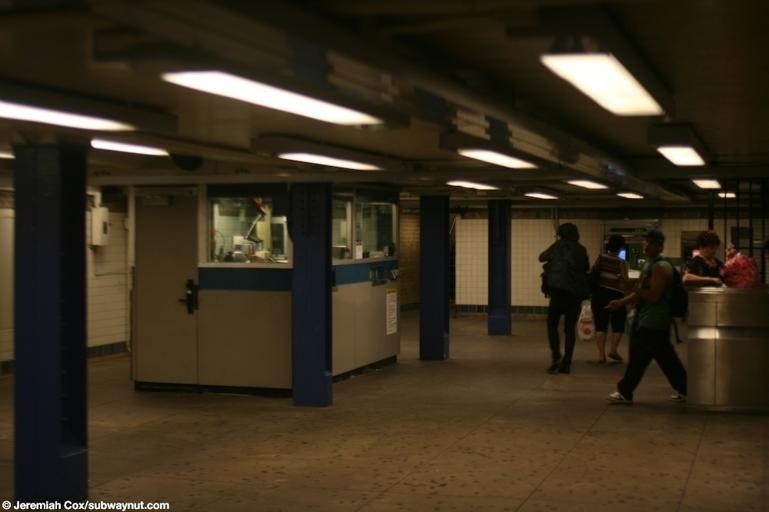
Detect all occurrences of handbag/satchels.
[577,299,596,341]
[720,248,759,289]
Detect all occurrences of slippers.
[608,352,622,361]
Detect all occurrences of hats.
[640,230,665,242]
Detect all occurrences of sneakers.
[670,394,686,402]
[605,392,634,404]
[547,361,571,373]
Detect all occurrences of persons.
[538,223,590,375]
[591,233,629,364]
[606,229,689,404]
[682,231,725,287]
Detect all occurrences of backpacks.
[647,257,688,321]
[540,257,573,299]
